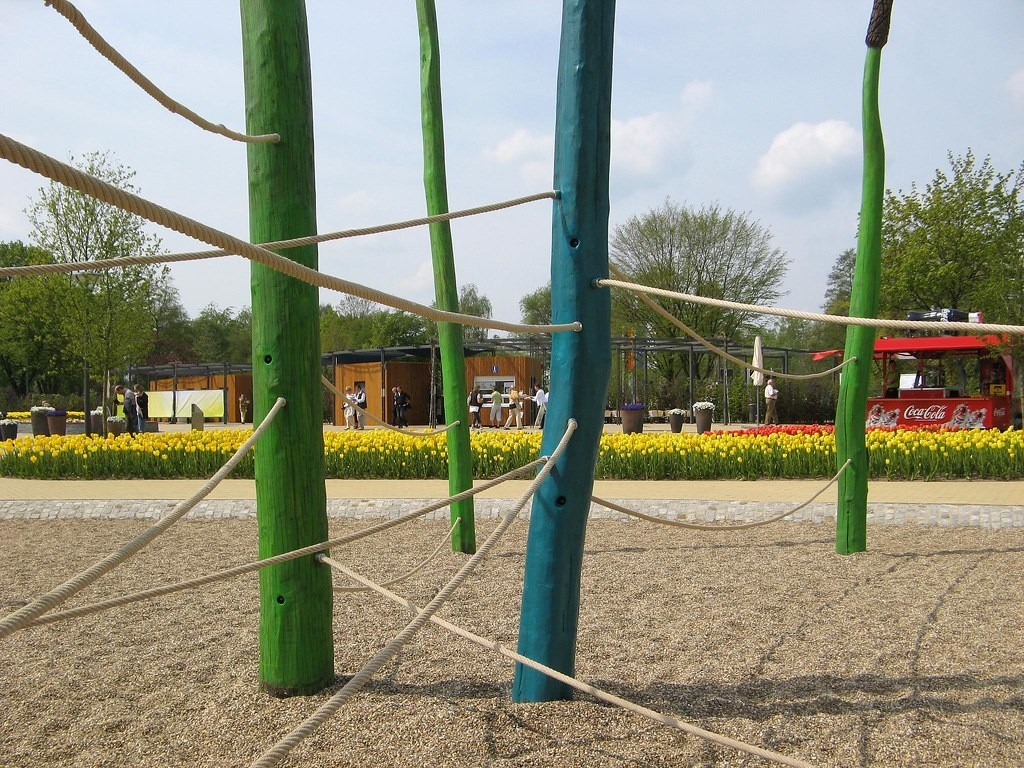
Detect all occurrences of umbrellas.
[749,336,764,425]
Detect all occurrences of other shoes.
[357,427,364,430]
[345,427,350,430]
[504,427,510,430]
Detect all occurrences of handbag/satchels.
[509,403,516,409]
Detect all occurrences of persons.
[765,379,782,426]
[391,386,411,428]
[884,360,900,398]
[134,384,148,433]
[239,394,248,424]
[343,385,367,430]
[489,387,502,428]
[504,385,550,430]
[115,385,137,433]
[467,385,483,431]
[1013,402,1022,430]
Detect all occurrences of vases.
[622,409,644,435]
[695,408,713,435]
[47,416,67,436]
[1,424,18,442]
[90,414,103,435]
[107,421,125,434]
[671,413,684,433]
[31,411,50,438]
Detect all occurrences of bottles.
[965,409,985,427]
[869,405,883,424]
[884,410,896,425]
[953,404,967,425]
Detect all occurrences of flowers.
[668,408,686,416]
[91,410,103,415]
[0,418,20,426]
[30,406,56,412]
[620,403,646,410]
[107,416,125,423]
[43,409,67,416]
[693,402,716,411]
